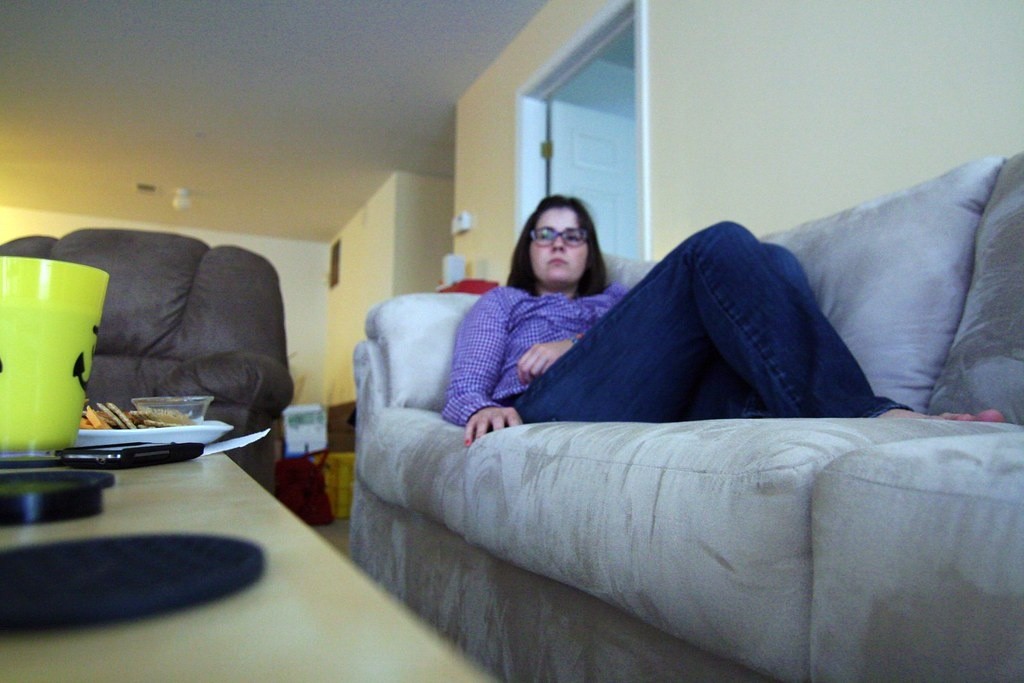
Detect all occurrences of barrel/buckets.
[0,254,111,454]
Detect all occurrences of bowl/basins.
[131,395,216,428]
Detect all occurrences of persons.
[441,194,1006,446]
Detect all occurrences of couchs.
[0,227,293,497]
[349,154,1022,682]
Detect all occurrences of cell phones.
[61,441,209,470]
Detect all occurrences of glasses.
[530,228,588,247]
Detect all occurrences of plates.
[72,418,235,447]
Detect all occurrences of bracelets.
[568,336,579,343]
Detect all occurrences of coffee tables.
[0,449,489,683]
